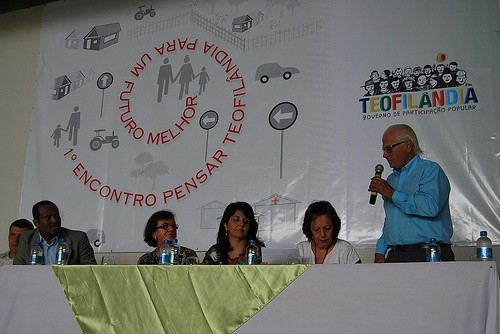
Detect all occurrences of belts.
[390,243,451,251]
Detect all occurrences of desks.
[0,260,500,334]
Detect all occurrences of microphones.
[370,164,384,204]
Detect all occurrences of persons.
[0,219,34,266]
[13,201,97,265]
[202,202,266,265]
[298,201,361,265]
[137,211,198,265]
[368,124,454,264]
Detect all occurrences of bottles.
[246,240,260,265]
[29,242,43,265]
[55,238,68,265]
[426,238,441,262]
[476,231,493,261]
[169,239,180,265]
[160,240,170,265]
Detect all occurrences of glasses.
[157,223,178,230]
[383,141,405,154]
